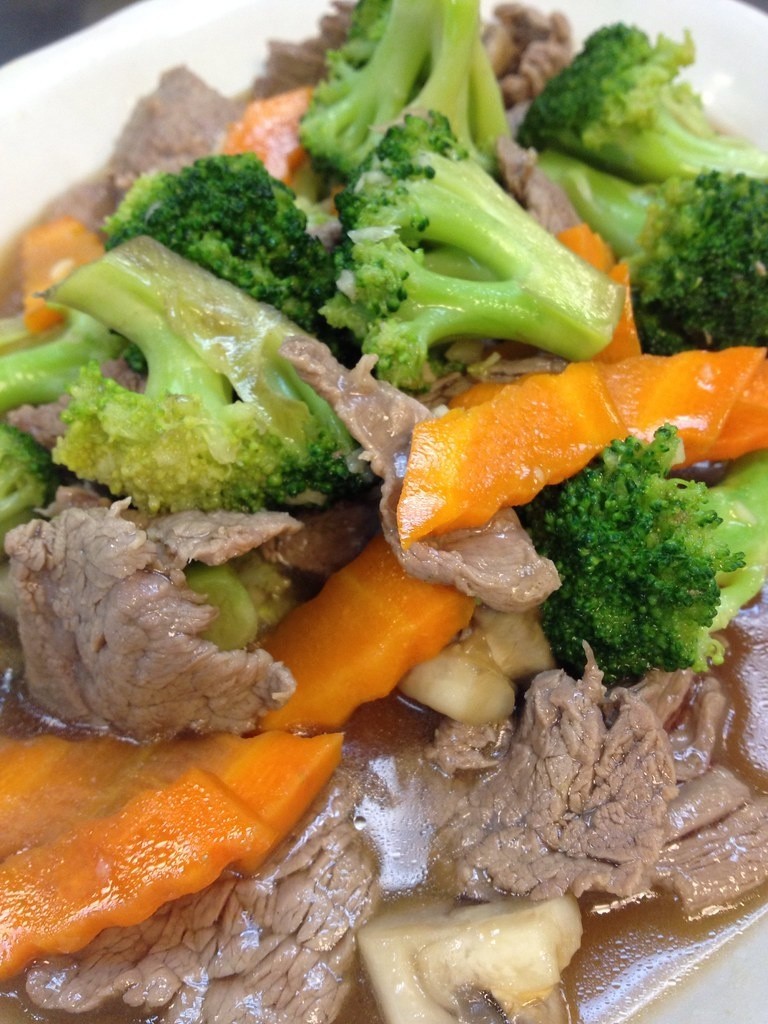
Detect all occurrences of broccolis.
[0,0,768,687]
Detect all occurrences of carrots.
[0,93,768,980]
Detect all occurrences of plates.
[0,1,768,1023]
